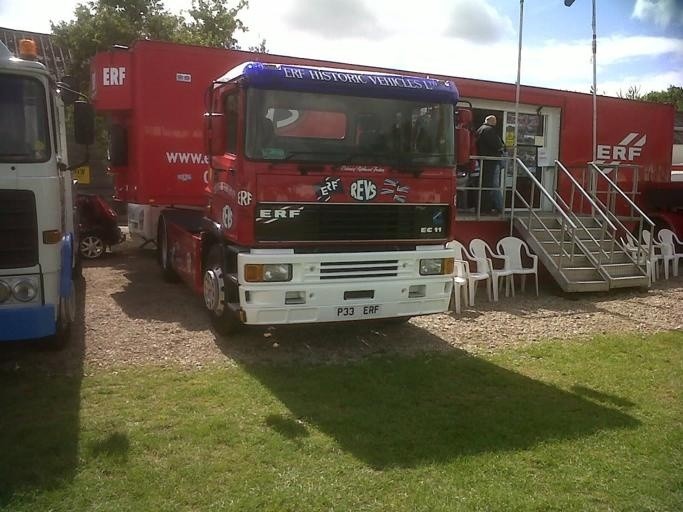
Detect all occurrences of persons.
[476,115,507,216]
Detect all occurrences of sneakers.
[463,206,500,217]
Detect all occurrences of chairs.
[619,233,656,287]
[446,239,492,307]
[416,244,468,314]
[469,238,515,301]
[658,229,683,276]
[497,237,538,296]
[642,230,677,280]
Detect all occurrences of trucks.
[0,33,85,345]
[155,60,478,335]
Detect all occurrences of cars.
[72,186,126,263]
